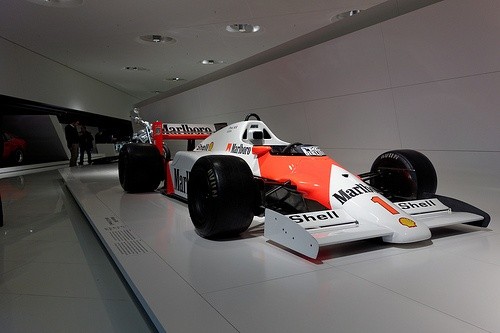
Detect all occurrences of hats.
[79,127,87,133]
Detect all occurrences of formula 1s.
[119,108,491,260]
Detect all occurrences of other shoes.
[87,161,92,165]
[78,162,83,165]
[70,164,76,167]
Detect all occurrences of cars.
[2,133,25,165]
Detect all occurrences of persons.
[95,127,105,144]
[78,124,94,166]
[65,118,80,167]
